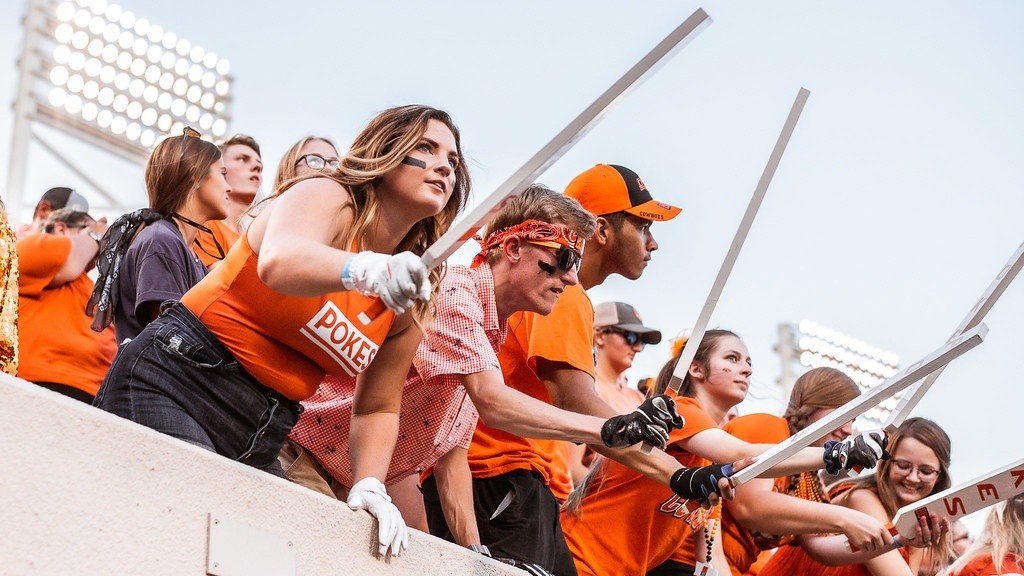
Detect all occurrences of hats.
[34,187,89,220]
[565,164,682,220]
[595,302,662,344]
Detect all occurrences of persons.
[1,104,1024,576]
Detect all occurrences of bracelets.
[893,535,902,548]
[342,255,354,290]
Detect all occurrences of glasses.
[526,239,582,274]
[180,127,201,157]
[892,458,942,482]
[296,154,339,171]
[953,531,969,543]
[604,328,647,348]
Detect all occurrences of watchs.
[88,231,101,243]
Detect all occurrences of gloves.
[341,250,431,315]
[347,476,407,557]
[601,394,684,451]
[823,429,891,475]
